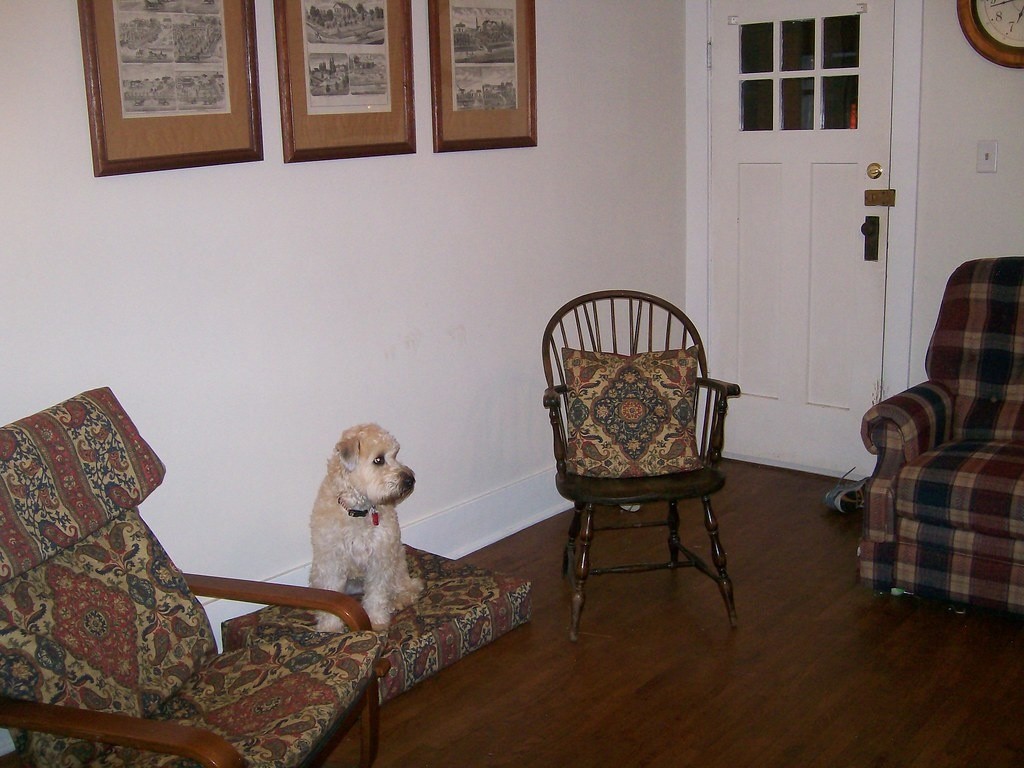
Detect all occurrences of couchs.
[857,257,1024,616]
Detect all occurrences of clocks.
[957,0,1024,68]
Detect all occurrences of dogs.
[308,423,426,632]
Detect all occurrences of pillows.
[557,345,703,477]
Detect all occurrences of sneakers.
[825,466,871,515]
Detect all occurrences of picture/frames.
[428,0,537,153]
[78,0,264,179]
[274,0,416,165]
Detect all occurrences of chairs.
[0,384,388,768]
[541,289,740,641]
